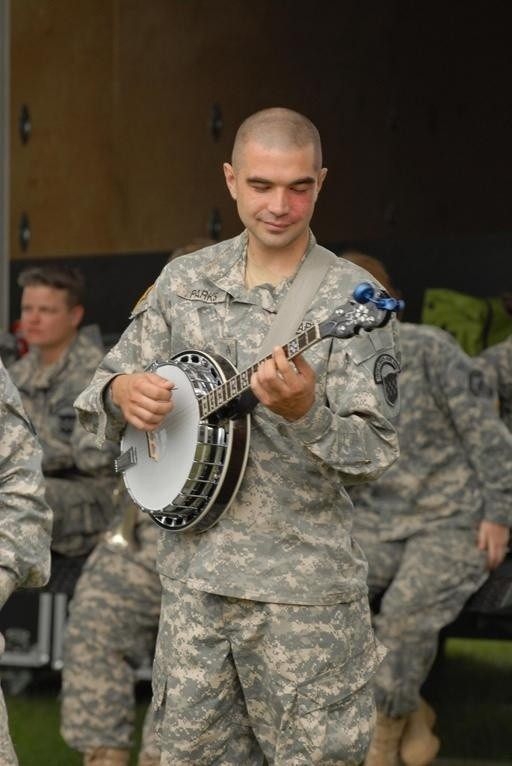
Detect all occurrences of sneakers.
[365,696,440,766]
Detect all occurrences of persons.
[338,248,512,766]
[57,497,162,766]
[468,332,512,614]
[0,357,58,766]
[9,260,117,558]
[66,104,406,766]
